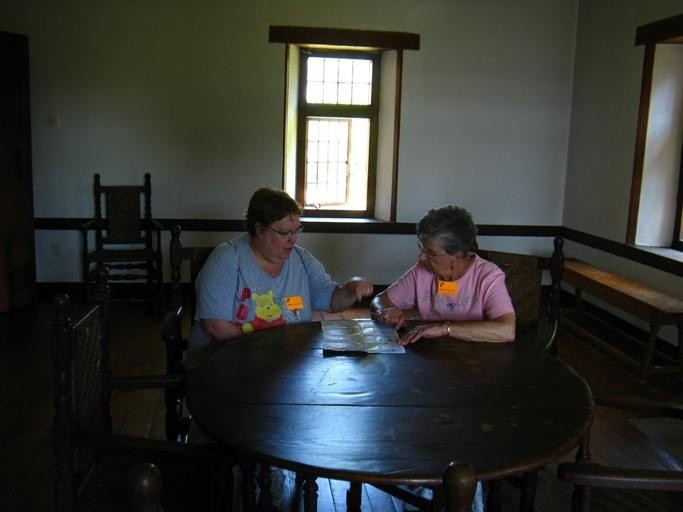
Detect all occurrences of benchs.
[556,257,681,384]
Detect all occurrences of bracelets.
[447,322,451,338]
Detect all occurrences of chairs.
[77,172,162,319]
[554,392,681,512]
[162,225,216,444]
[51,267,236,512]
[470,234,566,510]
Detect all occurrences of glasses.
[267,223,305,238]
[417,240,450,259]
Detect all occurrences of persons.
[184,189,374,372]
[370,206,516,511]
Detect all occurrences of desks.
[183,317,596,512]
